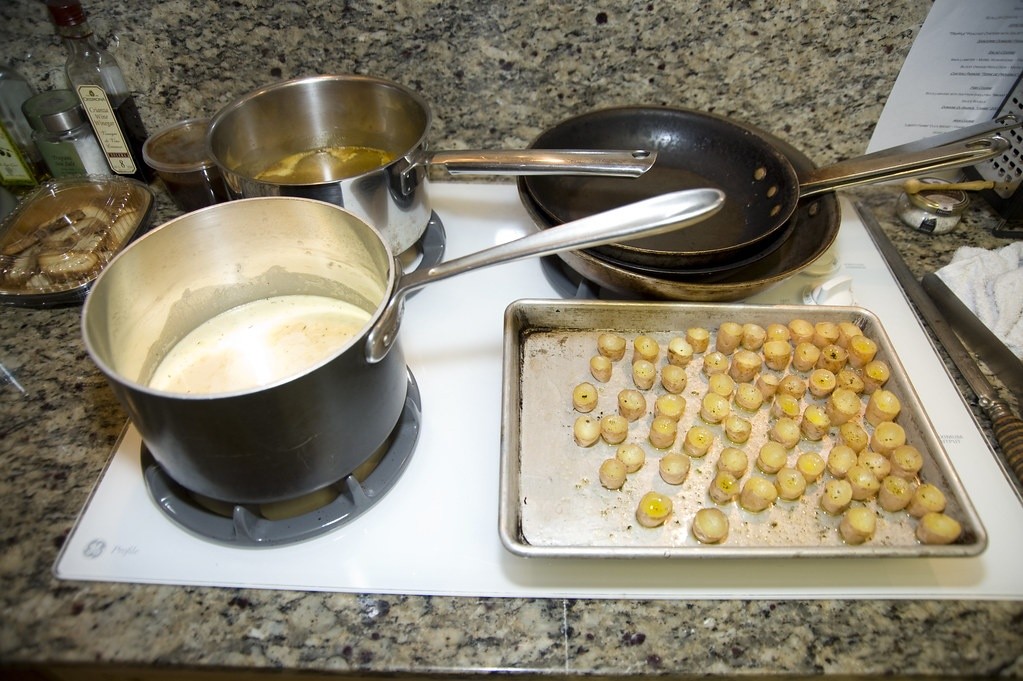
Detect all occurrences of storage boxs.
[0,169,158,309]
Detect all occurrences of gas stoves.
[49,155,1023,602]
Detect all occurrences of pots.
[205,74,658,290]
[513,105,1022,303]
[82,186,725,505]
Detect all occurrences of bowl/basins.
[142,115,227,213]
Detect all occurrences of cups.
[896,177,968,234]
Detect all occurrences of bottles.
[22,89,113,177]
[45,0,156,182]
[1,67,52,189]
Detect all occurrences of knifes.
[918,275,1023,407]
